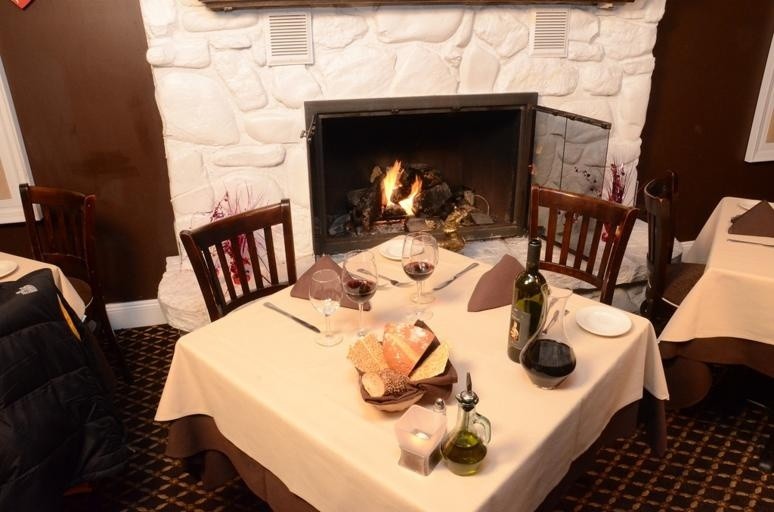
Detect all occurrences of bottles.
[507,239,551,363]
[520,282,579,390]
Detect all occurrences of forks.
[355,268,412,288]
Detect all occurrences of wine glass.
[307,267,346,348]
[343,249,379,339]
[400,231,440,322]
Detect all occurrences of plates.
[571,305,631,339]
[381,240,425,262]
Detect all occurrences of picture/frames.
[0,65,42,225]
[743,34,774,164]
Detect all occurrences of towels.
[728,201,773,248]
[467,253,539,311]
[289,255,375,313]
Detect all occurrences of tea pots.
[440,369,494,476]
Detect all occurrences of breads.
[347,320,450,397]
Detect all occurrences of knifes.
[263,300,321,333]
[430,261,481,290]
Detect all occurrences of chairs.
[638,168,705,330]
[18,181,132,384]
[179,196,299,321]
[525,182,641,304]
